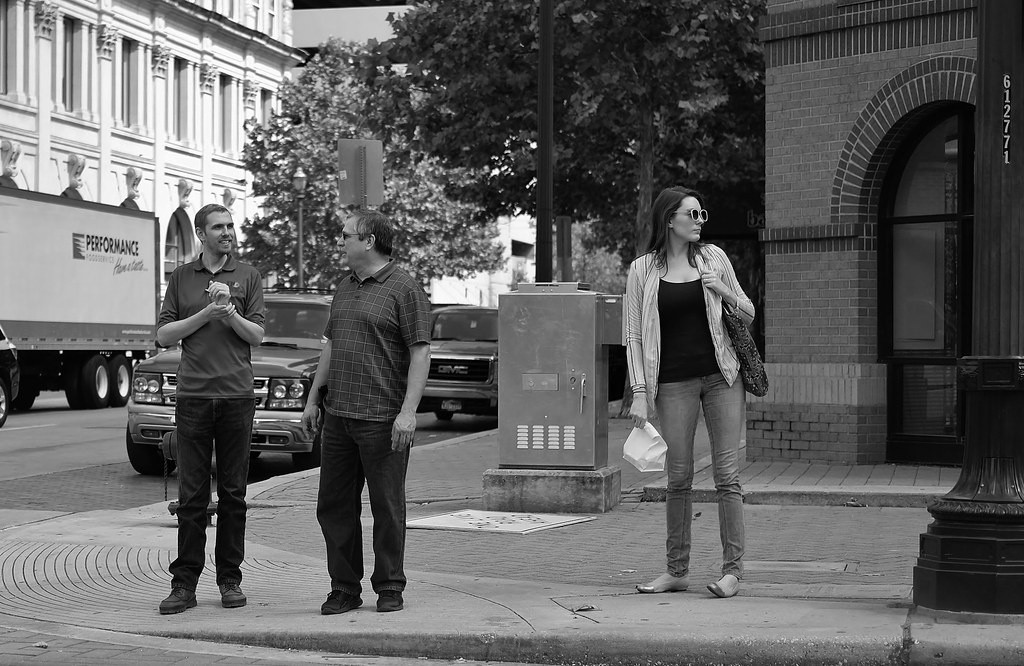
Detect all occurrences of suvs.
[125,288,335,479]
[415,304,497,420]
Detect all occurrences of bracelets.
[632,386,647,396]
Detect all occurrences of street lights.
[294,167,308,294]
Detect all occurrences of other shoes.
[635,583,688,592]
[706,574,739,598]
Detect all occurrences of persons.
[301,207,433,616]
[156,204,266,615]
[625,184,756,599]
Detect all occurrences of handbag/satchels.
[621,420,667,473]
[720,299,769,398]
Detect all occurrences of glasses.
[342,232,371,240]
[671,209,709,223]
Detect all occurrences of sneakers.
[321,590,363,614]
[219,583,247,608]
[376,590,404,612]
[159,587,197,615]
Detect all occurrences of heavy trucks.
[0,185,160,418]
